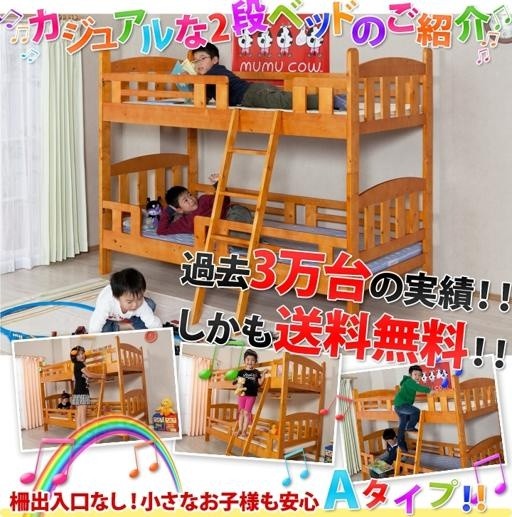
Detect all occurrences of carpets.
[1,274,323,360]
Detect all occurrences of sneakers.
[405,426,417,432]
[399,444,408,453]
[237,431,248,437]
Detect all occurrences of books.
[171,58,197,103]
[367,460,394,474]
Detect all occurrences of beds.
[92,42,435,336]
[32,337,150,443]
[203,350,328,464]
[350,371,505,479]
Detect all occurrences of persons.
[233,349,268,437]
[87,268,162,334]
[58,393,72,415]
[234,376,247,397]
[181,42,348,110]
[71,346,106,430]
[370,428,408,478]
[393,365,438,453]
[157,173,269,253]
